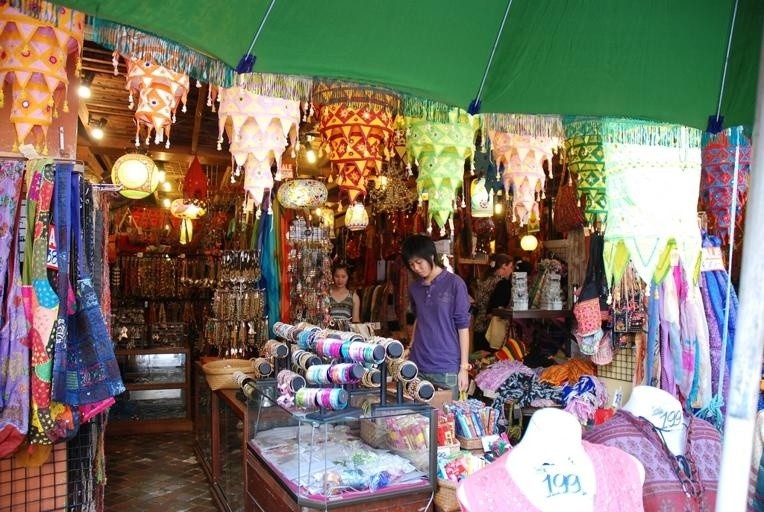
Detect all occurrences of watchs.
[459,364,472,370]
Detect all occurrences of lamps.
[77,70,95,99]
[87,114,108,140]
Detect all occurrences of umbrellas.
[47,0,764,511]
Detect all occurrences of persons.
[472,252,513,352]
[327,263,361,323]
[454,406,646,512]
[582,383,722,512]
[400,234,471,402]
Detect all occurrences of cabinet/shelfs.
[103,345,194,436]
[192,357,306,512]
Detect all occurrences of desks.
[496,311,571,358]
